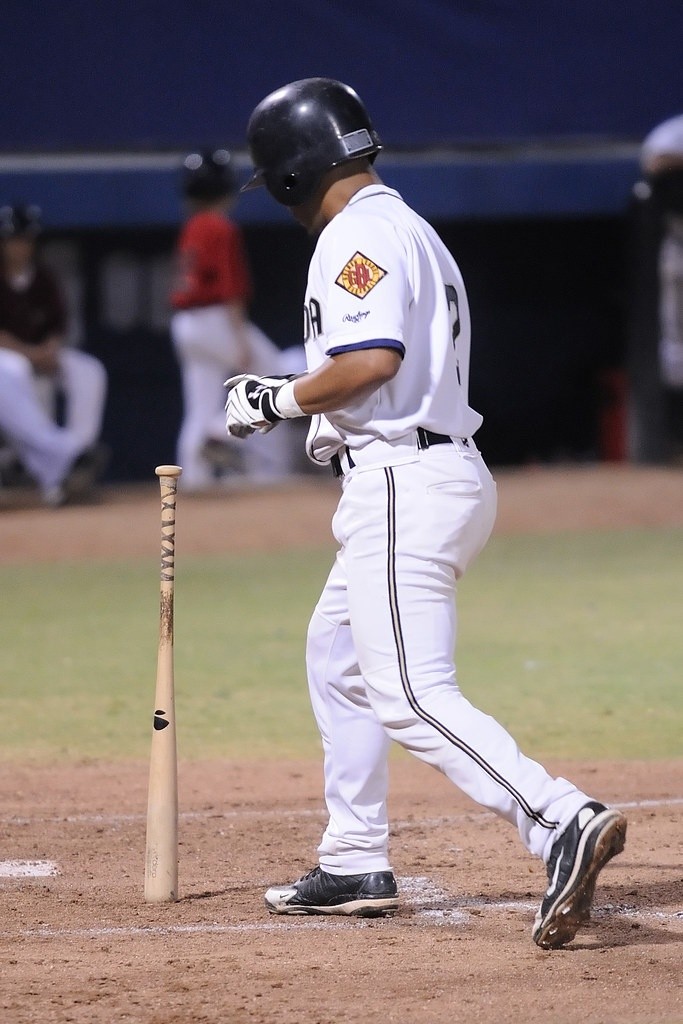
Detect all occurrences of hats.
[0,202,41,244]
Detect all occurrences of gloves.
[223,369,308,440]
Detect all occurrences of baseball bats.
[142,465,182,902]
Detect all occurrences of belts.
[330,428,468,480]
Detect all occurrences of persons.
[167,148,292,489]
[0,203,108,505]
[640,110,683,448]
[223,74,627,946]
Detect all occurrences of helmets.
[238,77,381,207]
[178,150,238,198]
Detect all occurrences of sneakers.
[531,802,627,950]
[265,866,399,916]
